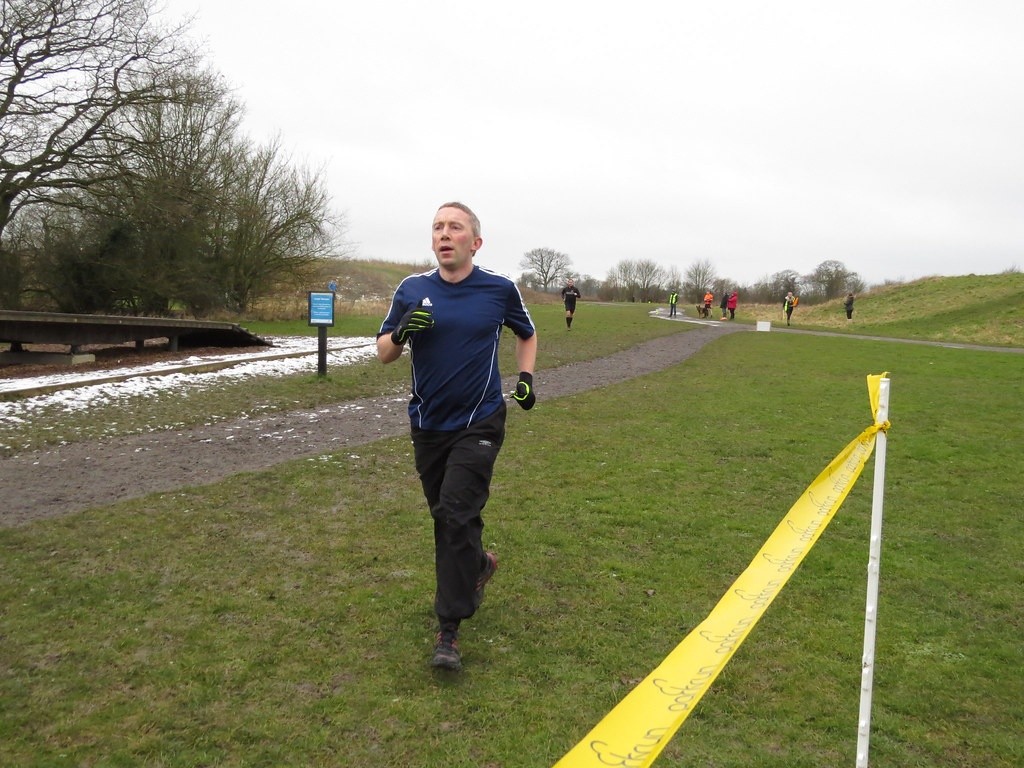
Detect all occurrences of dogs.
[696,305,709,318]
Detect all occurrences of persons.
[561,279,581,331]
[844,292,854,319]
[719,291,738,320]
[783,291,796,326]
[377,203,538,669]
[703,290,713,319]
[668,291,677,317]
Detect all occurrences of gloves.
[509,372,535,410]
[391,300,435,345]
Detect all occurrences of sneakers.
[472,552,496,611]
[429,629,462,671]
